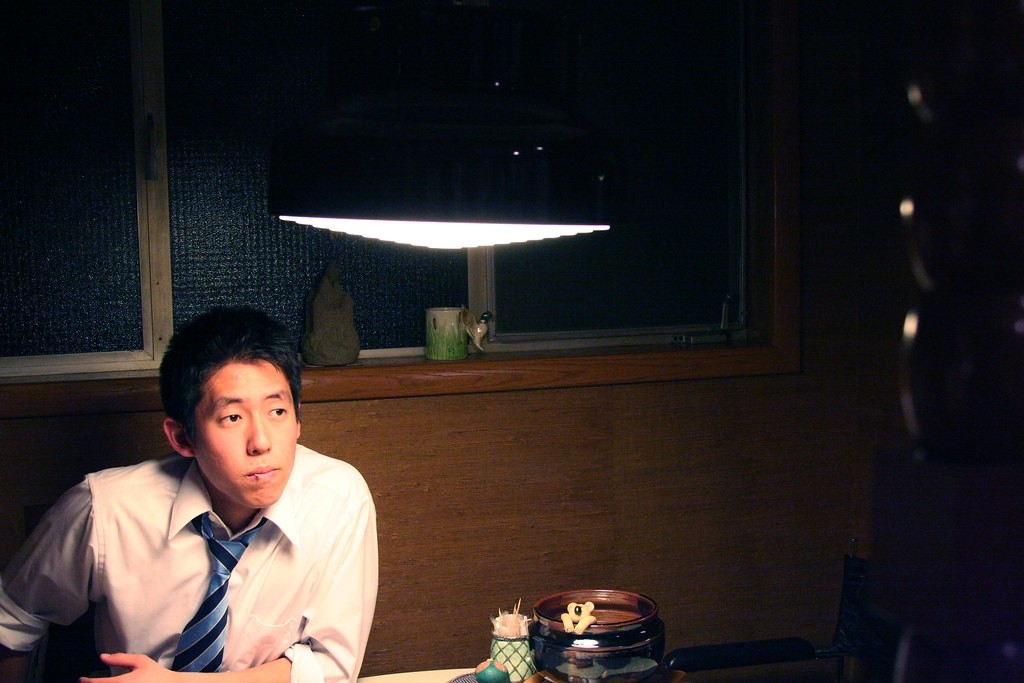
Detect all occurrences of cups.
[426,307,466,360]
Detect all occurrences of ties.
[171,512,269,673]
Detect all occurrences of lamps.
[268,0,627,227]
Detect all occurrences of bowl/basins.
[526,589,666,683]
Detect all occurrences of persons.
[0,305,380,683]
